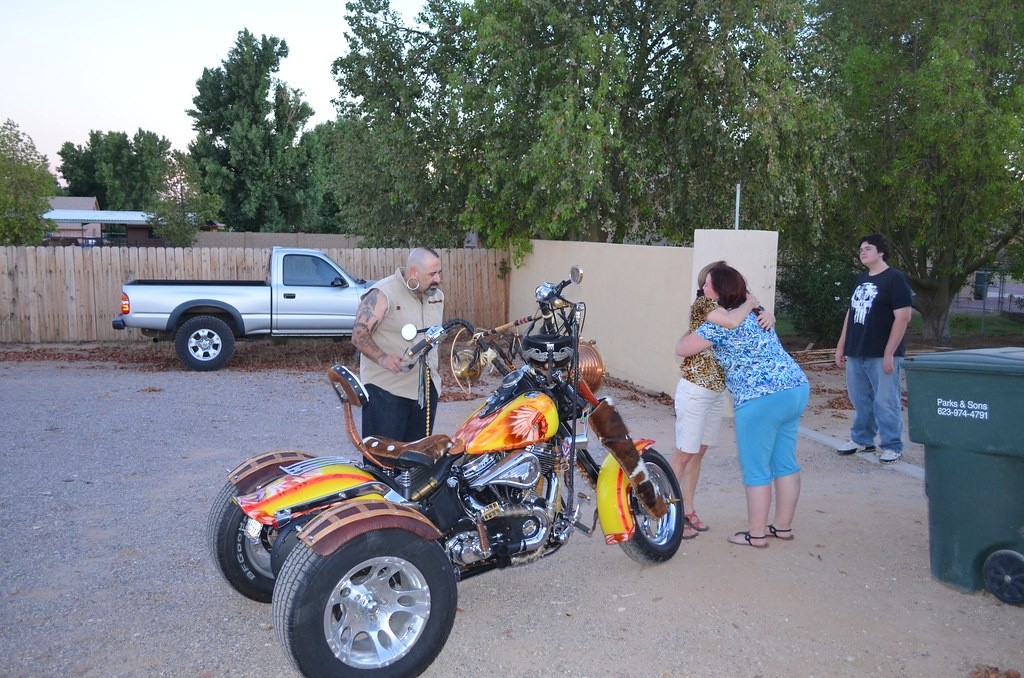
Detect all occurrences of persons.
[834,233,912,463]
[667,260,777,540]
[351,245,444,472]
[674,265,810,547]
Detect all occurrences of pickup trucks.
[113,245,376,372]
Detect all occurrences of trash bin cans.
[898,347,1024,606]
[975,273,993,300]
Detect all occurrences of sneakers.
[880,449,902,463]
[837,439,877,454]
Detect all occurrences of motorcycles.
[207,263,683,678]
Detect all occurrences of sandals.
[682,523,699,539]
[728,529,768,548]
[683,510,708,531]
[764,523,794,541]
